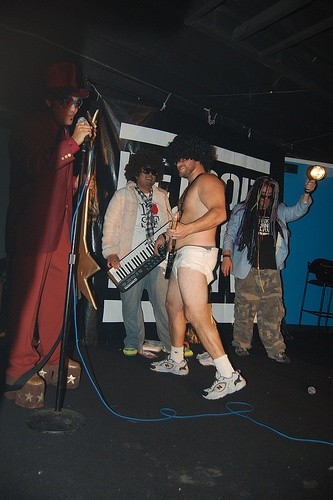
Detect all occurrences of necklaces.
[178,172,206,222]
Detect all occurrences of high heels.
[0,369,45,409]
[35,357,81,390]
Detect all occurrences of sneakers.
[161,345,194,359]
[201,369,247,401]
[267,352,290,363]
[148,355,190,376]
[122,347,138,357]
[235,346,249,356]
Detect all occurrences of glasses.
[52,96,83,110]
[260,194,272,199]
[143,167,159,177]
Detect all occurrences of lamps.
[306,165,327,180]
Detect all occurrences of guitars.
[77,109,101,310]
[164,206,180,280]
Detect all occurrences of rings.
[172,236,173,237]
[88,129,90,132]
[84,120,87,126]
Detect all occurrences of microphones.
[78,117,92,153]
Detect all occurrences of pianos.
[108,220,176,293]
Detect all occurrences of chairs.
[298,258,333,338]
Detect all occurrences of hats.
[36,61,90,98]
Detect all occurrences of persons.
[102,149,194,358]
[0,63,93,409]
[221,176,317,365]
[149,133,246,400]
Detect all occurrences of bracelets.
[304,190,312,194]
[223,255,231,257]
[161,233,167,243]
